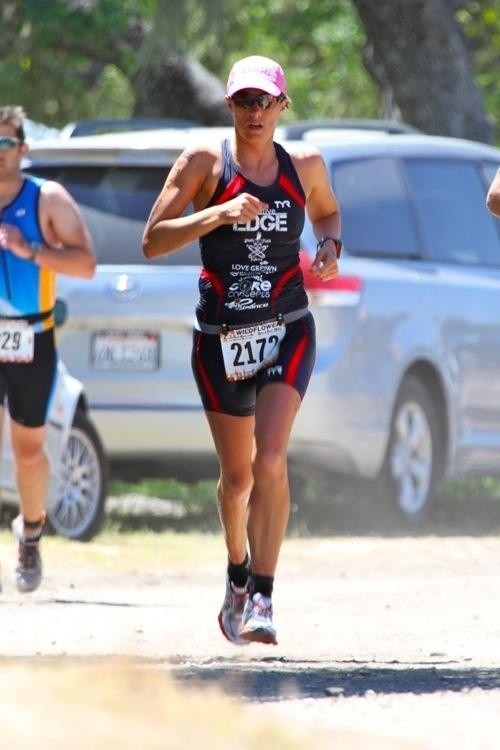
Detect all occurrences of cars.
[0,355,110,544]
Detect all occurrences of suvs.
[13,103,498,540]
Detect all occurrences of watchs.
[318,235,343,258]
[23,237,44,265]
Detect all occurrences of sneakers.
[218,559,277,644]
[14,516,41,590]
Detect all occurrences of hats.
[226,55,287,96]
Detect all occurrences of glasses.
[0,136,23,149]
[233,92,274,110]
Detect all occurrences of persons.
[138,54,343,645]
[484,164,500,222]
[0,103,95,593]
